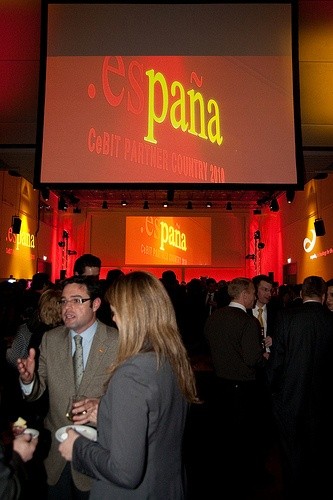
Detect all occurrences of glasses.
[56,298,94,306]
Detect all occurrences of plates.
[55,425,97,442]
[23,427,39,439]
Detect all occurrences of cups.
[65,395,87,422]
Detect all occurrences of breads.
[12,417,27,434]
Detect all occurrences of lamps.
[285,190,294,204]
[206,202,212,208]
[163,202,168,207]
[253,208,261,214]
[121,201,127,206]
[41,190,49,201]
[186,201,192,209]
[74,207,81,213]
[143,201,149,209]
[102,201,108,209]
[226,202,232,210]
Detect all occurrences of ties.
[258,308,263,328]
[72,335,84,392]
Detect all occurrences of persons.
[0,433,38,500]
[263,276,333,500]
[16,276,121,500]
[0,271,232,417]
[71,254,101,290]
[203,278,271,483]
[248,275,284,374]
[58,270,201,500]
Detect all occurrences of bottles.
[261,326,266,353]
